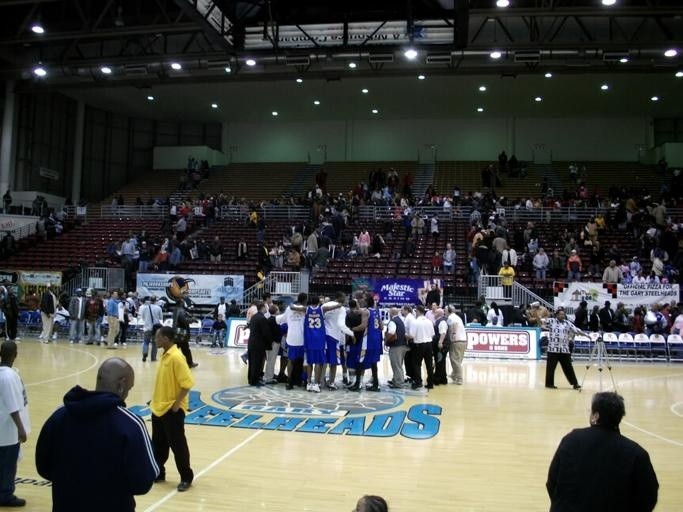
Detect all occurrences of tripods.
[580,336,617,395]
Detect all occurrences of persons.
[0,274,240,370]
[105,154,232,287]
[232,161,452,271]
[0,179,88,258]
[452,148,683,336]
[538,311,583,390]
[0,341,29,507]
[546,391,659,512]
[351,495,388,512]
[242,281,467,392]
[146,327,194,492]
[35,357,161,511]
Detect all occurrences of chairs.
[540,330,683,364]
[0,311,222,347]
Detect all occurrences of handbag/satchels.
[175,328,187,342]
[153,324,162,335]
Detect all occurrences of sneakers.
[2,494,26,507]
[6,334,198,368]
[177,480,190,491]
[155,473,165,481]
[241,354,463,393]
